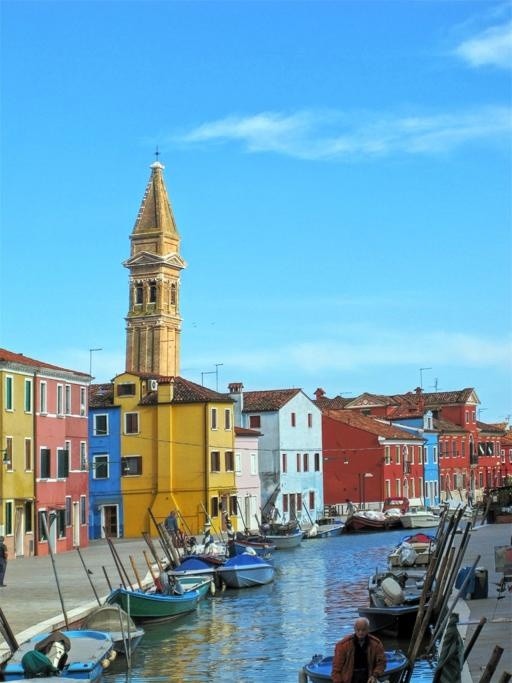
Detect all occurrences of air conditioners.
[147,378,159,391]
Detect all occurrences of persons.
[346,498,359,515]
[466,488,473,507]
[332,617,386,683]
[267,505,281,524]
[0,536,8,587]
[165,511,179,535]
